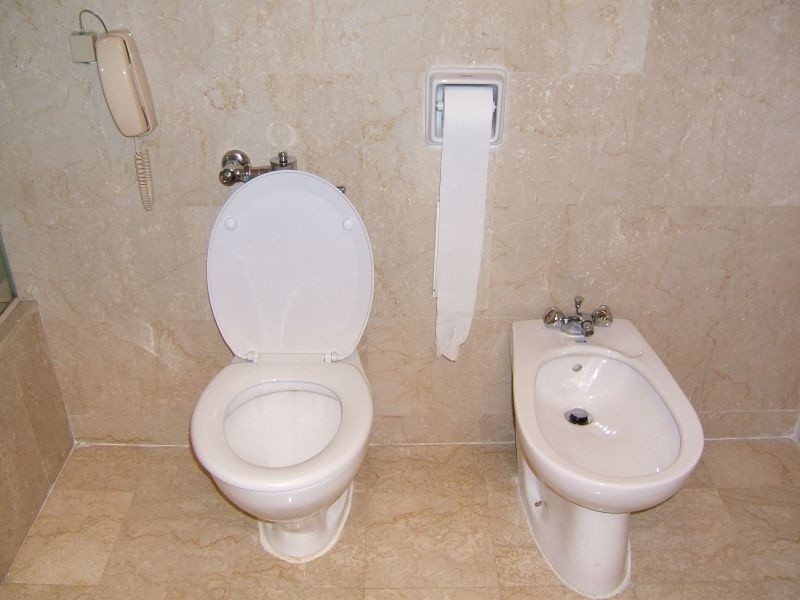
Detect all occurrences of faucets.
[541,291,615,337]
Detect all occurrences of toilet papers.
[431,84,497,363]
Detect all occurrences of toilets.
[188,168,378,563]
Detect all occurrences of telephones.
[96,30,156,136]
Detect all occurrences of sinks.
[509,315,708,598]
